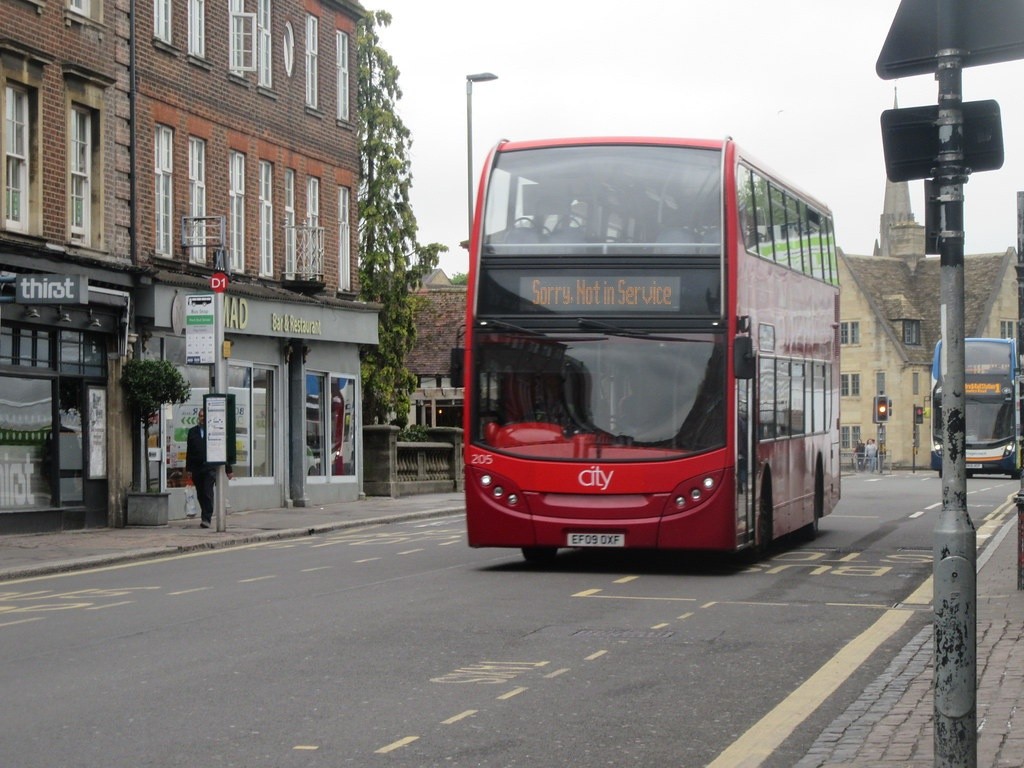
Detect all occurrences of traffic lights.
[875,395,890,423]
[914,406,923,425]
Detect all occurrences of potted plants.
[117,356,193,527]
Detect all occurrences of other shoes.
[200,518,211,528]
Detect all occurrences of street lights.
[465,71,500,237]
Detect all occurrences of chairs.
[595,236,625,254]
[579,224,600,254]
[532,224,554,244]
[551,216,587,254]
[506,215,546,253]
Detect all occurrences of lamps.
[87,318,102,327]
[26,308,40,318]
[303,343,312,364]
[57,313,72,323]
[283,340,294,364]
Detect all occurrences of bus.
[450,135,844,575]
[922,337,1021,481]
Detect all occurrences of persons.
[632,364,689,427]
[186,409,233,527]
[855,439,877,473]
